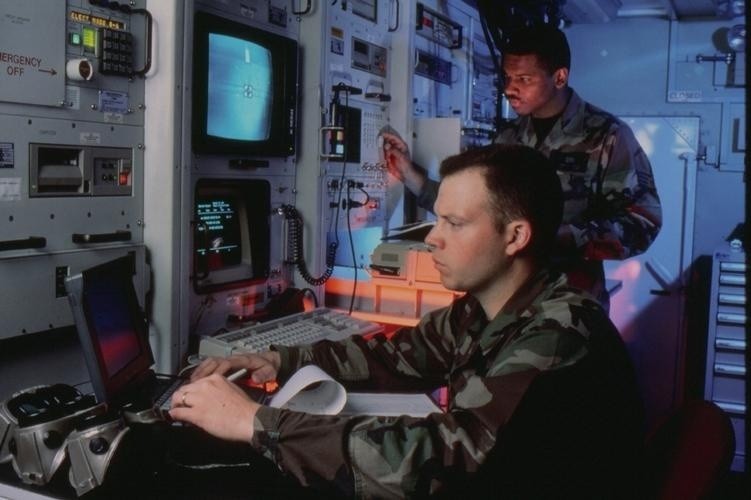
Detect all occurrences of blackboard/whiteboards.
[0,0,67,109]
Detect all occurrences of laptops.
[61,253,267,435]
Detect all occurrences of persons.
[377,23,663,319]
[168,143,645,500]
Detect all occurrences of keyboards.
[197,306,388,363]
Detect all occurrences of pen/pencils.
[225,368,247,381]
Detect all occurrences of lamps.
[727,24,746,87]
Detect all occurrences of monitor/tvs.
[198,21,299,159]
[194,190,258,283]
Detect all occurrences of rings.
[182,392,188,407]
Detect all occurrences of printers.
[369,241,442,292]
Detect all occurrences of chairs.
[633,399,736,500]
